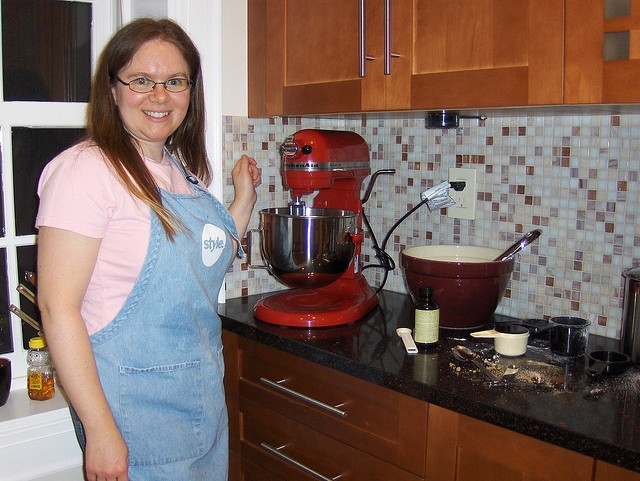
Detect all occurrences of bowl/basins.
[402,245,515,330]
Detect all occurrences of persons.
[35,15,263,480]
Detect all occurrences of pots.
[532,315,590,356]
[469,323,530,357]
[584,350,631,378]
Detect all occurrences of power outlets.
[446,167,476,220]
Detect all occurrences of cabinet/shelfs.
[222,330,424,481]
[423,387,640,481]
[566,0,639,105]
[246,1,565,119]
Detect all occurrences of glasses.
[117,77,195,93]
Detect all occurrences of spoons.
[396,327,419,354]
[452,344,501,385]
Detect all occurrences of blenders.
[245,127,380,329]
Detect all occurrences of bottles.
[410,287,441,350]
